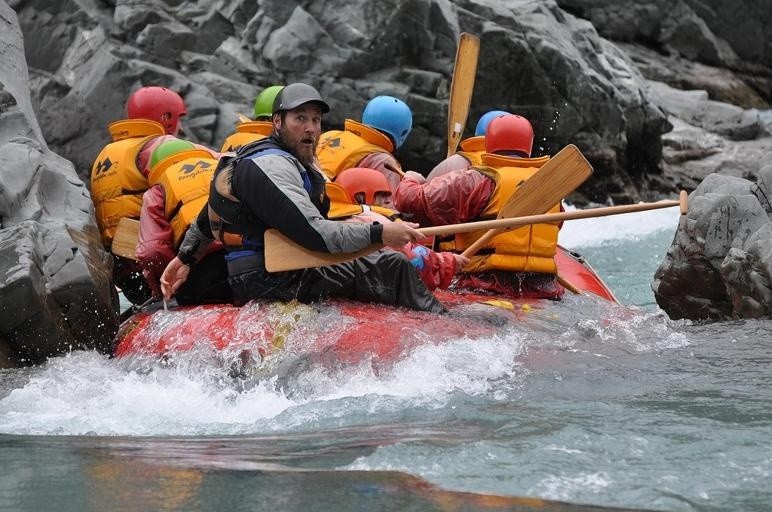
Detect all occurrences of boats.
[112,244,629,378]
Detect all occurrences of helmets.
[475,109,535,157]
[254,85,285,121]
[361,94,413,151]
[271,82,331,118]
[334,167,393,204]
[127,86,188,134]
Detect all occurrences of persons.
[394,113,567,301]
[89,84,285,307]
[207,84,449,313]
[314,94,505,292]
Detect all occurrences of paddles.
[432,32,481,253]
[265,191,687,273]
[460,143,595,258]
[110,217,141,259]
